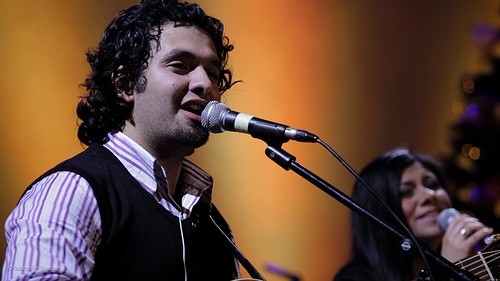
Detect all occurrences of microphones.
[437,208,497,251]
[200,101,319,143]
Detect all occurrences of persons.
[331,148,500,280]
[0,1,240,281]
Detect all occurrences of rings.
[460,228,468,237]
